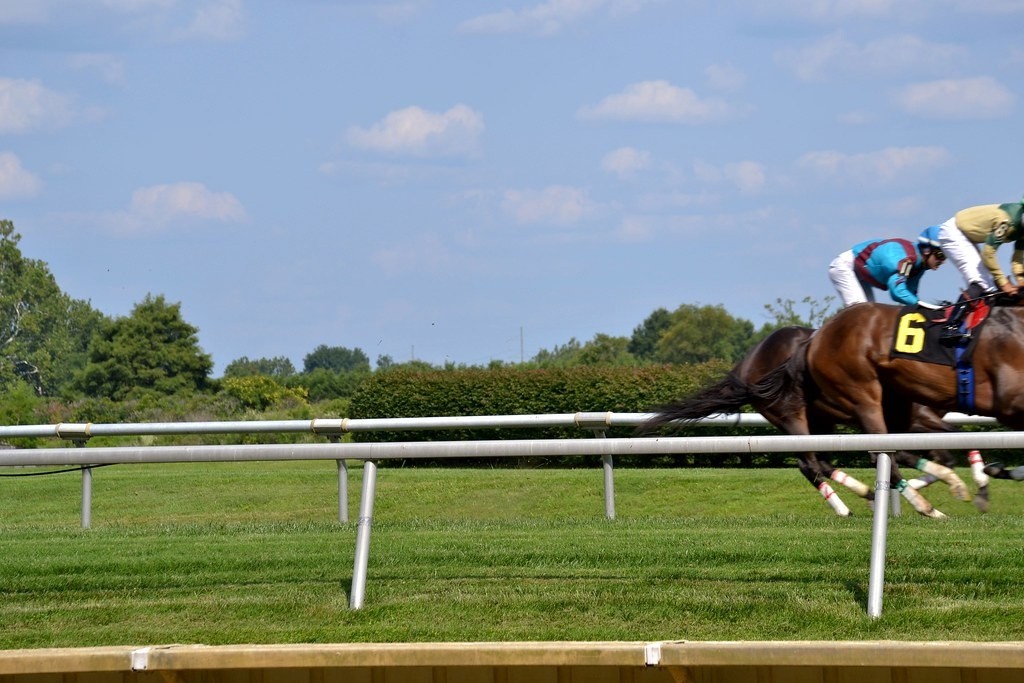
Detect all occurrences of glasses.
[929,245,946,261]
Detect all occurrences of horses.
[627,301,1023,524]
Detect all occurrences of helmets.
[917,225,943,247]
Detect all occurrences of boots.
[939,293,975,345]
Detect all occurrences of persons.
[937,197,1024,340]
[828,224,948,310]
